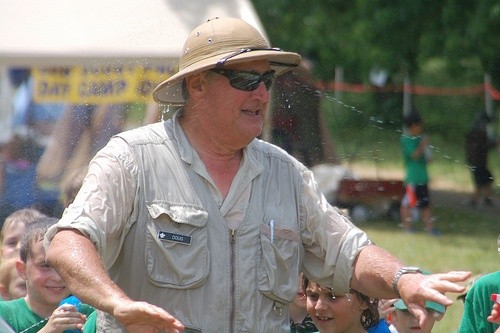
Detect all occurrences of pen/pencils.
[267,219,276,245]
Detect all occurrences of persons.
[0,208,98,333]
[290,265,500,333]
[400,111,442,237]
[463,109,500,209]
[43,17,472,333]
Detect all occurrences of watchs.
[392,265,422,299]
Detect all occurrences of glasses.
[211,68,281,92]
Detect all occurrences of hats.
[152,15,305,113]
